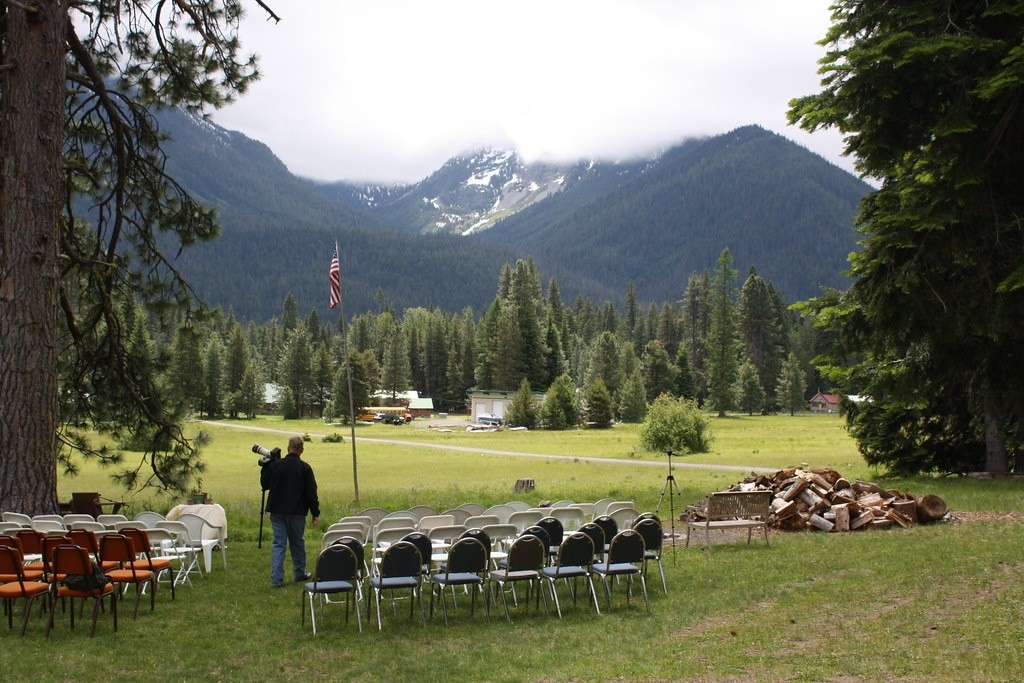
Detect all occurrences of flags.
[329,240,341,309]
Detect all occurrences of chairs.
[301,498,667,635]
[0,492,226,641]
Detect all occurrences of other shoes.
[301,572,311,581]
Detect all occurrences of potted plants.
[186,479,213,505]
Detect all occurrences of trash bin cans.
[72,492,103,522]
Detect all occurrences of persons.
[260,436,320,588]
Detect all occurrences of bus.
[354,406,412,422]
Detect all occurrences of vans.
[373,413,385,419]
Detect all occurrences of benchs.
[685,490,773,550]
[168,504,227,544]
